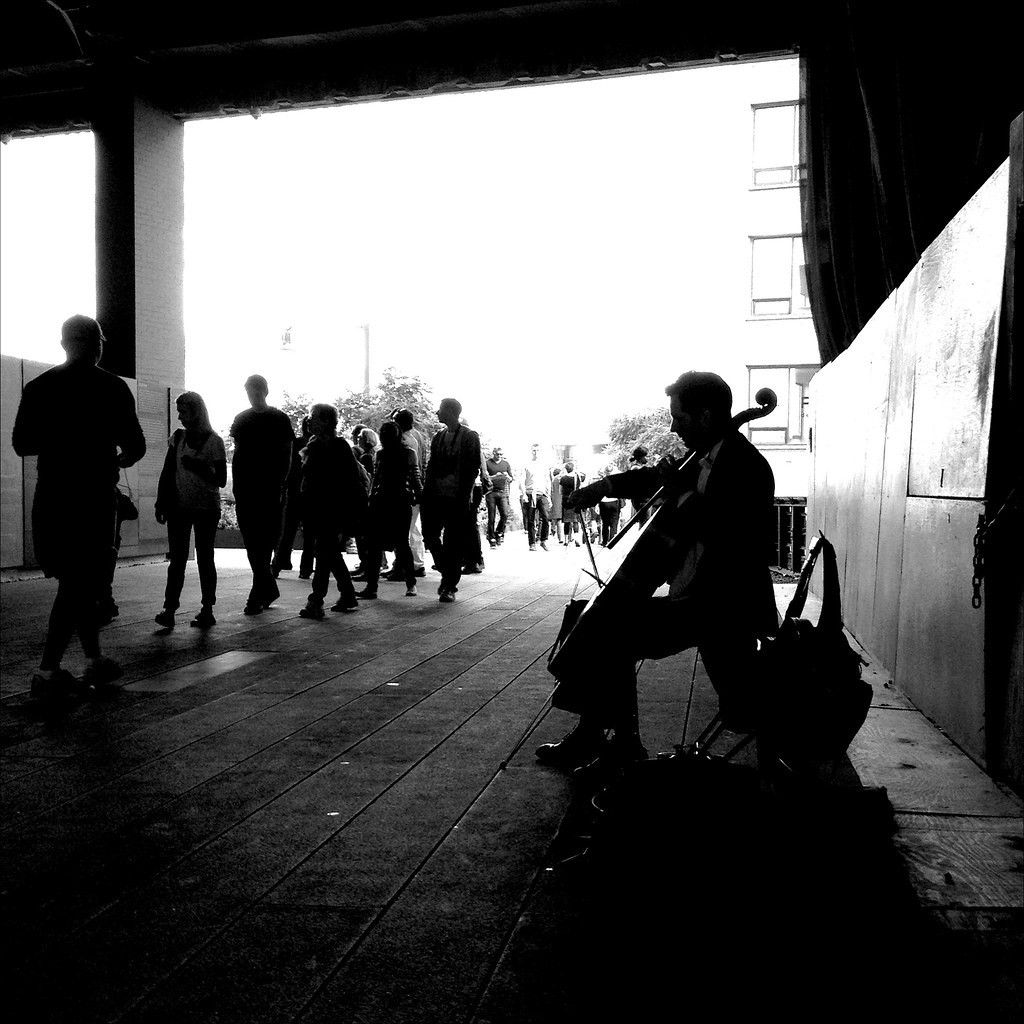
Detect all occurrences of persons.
[11,316,148,672]
[154,375,659,636]
[535,373,776,767]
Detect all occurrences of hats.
[61,314,107,342]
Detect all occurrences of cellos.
[498,383,781,771]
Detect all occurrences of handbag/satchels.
[702,538,868,734]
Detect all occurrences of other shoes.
[357,589,378,599]
[463,563,482,574]
[490,541,497,549]
[272,556,293,576]
[331,594,359,611]
[529,543,536,552]
[541,540,551,552]
[407,586,418,596]
[300,601,326,619]
[591,532,599,543]
[537,722,602,769]
[498,537,504,542]
[437,588,456,602]
[190,609,216,627]
[415,567,426,577]
[382,569,400,580]
[244,589,280,615]
[83,657,124,683]
[155,611,175,628]
[572,735,649,795]
[351,576,369,582]
[31,668,73,697]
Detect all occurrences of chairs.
[687,539,824,779]
